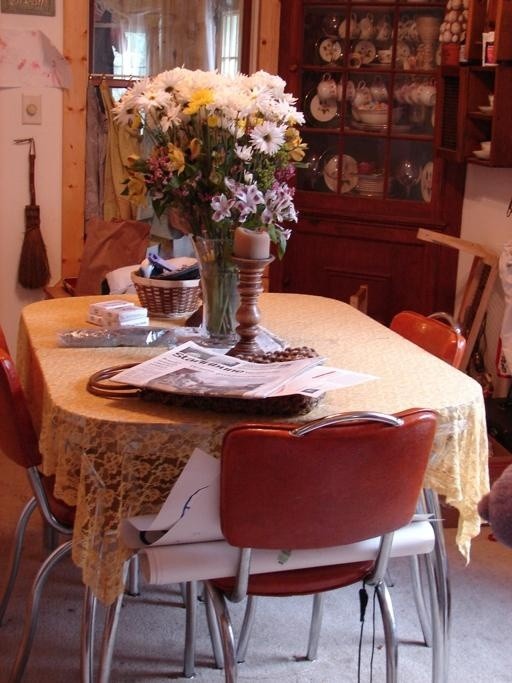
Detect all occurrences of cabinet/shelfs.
[273,1,511,372]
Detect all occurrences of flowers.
[107,61,308,340]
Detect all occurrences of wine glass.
[392,160,422,199]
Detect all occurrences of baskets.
[130,271,200,318]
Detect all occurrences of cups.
[320,11,420,42]
[318,72,439,106]
[377,50,397,64]
[415,15,441,68]
[480,142,491,152]
[489,94,494,107]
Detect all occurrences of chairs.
[205,407,443,682]
[1,329,201,682]
[237,311,466,680]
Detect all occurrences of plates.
[472,150,491,160]
[353,42,378,65]
[312,148,395,199]
[420,160,433,203]
[301,86,341,129]
[350,121,415,134]
[479,106,494,112]
[319,38,342,63]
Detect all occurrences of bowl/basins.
[347,53,362,69]
[355,109,404,124]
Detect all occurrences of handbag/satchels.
[87,346,325,415]
[74,218,151,297]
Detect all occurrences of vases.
[192,233,242,343]
